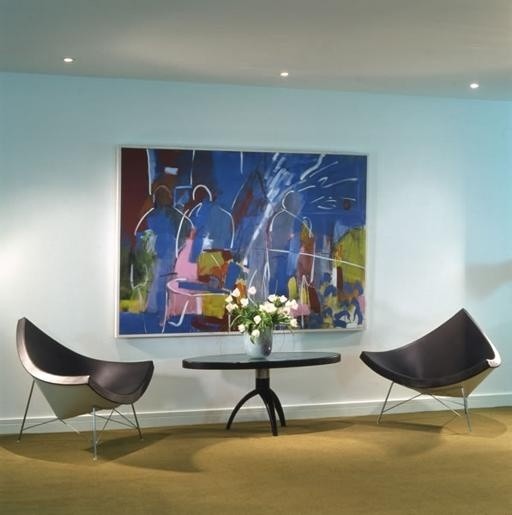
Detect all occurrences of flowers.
[224,283,303,340]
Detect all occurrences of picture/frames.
[111,142,372,340]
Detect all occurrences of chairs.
[357,307,502,431]
[12,313,155,462]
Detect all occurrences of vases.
[244,320,275,359]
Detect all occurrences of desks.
[182,347,345,443]
